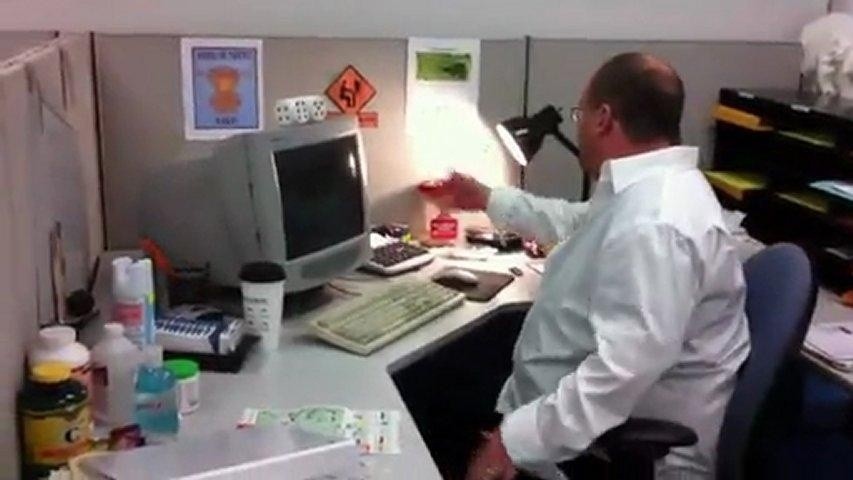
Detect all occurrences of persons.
[416,51,755,478]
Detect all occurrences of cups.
[238,260,287,352]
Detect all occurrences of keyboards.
[309,278,467,356]
[362,242,437,276]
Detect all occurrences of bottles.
[18,257,202,480]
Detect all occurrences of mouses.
[434,269,479,285]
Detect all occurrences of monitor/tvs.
[137,115,374,318]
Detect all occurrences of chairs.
[465,241,818,480]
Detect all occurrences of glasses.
[570,106,584,122]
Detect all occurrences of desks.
[87,233,852,480]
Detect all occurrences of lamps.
[494,104,589,201]
